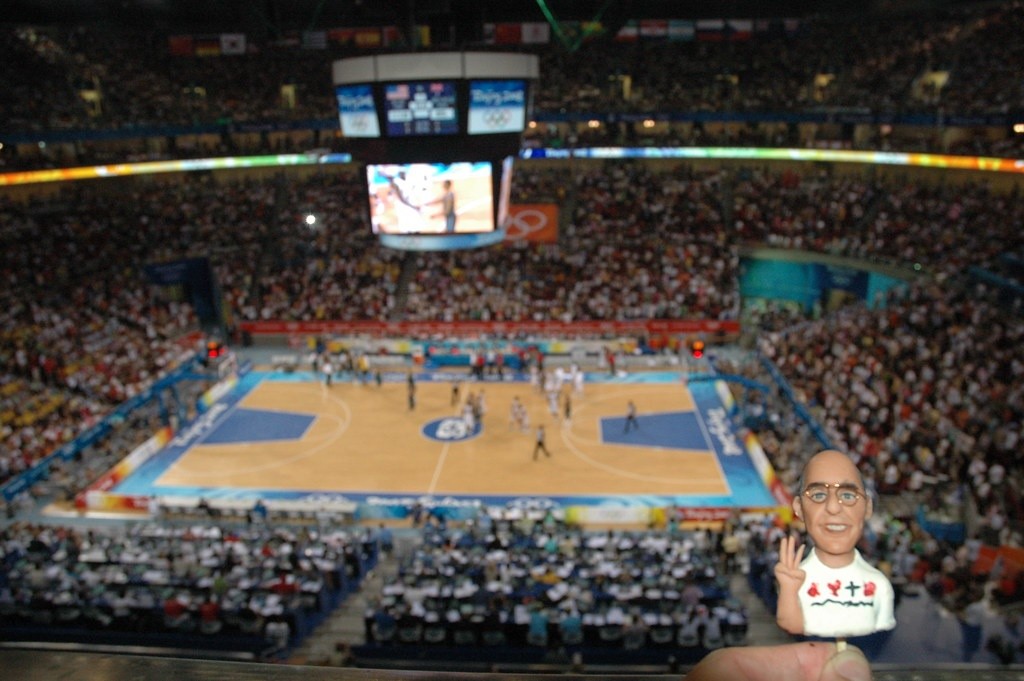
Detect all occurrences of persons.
[424,180,456,233]
[774,448,897,638]
[1,0,1024,660]
[684,643,873,681]
[393,172,417,231]
[373,173,419,232]
